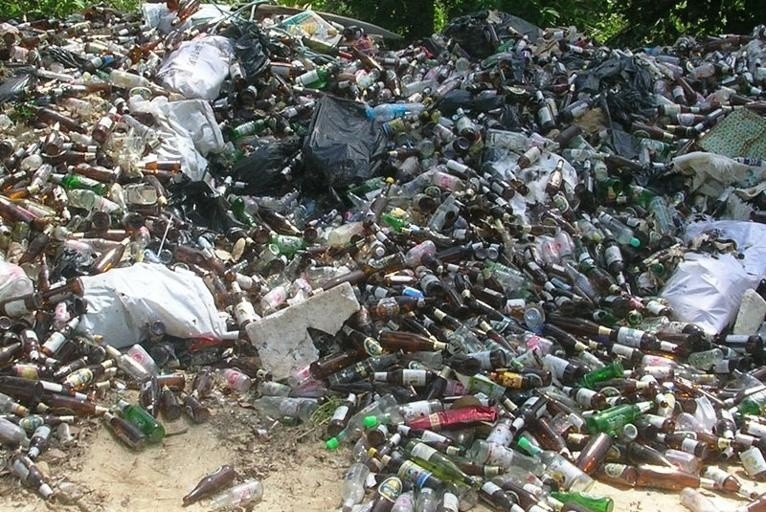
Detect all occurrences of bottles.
[5,449,55,499]
[608,24,766,151]
[327,401,766,512]
[178,1,408,275]
[0,275,103,450]
[409,138,611,280]
[208,482,262,507]
[211,278,349,423]
[409,12,607,136]
[0,1,177,274]
[98,407,144,448]
[116,397,167,442]
[112,324,218,422]
[351,280,766,400]
[182,465,235,502]
[613,151,735,288]
